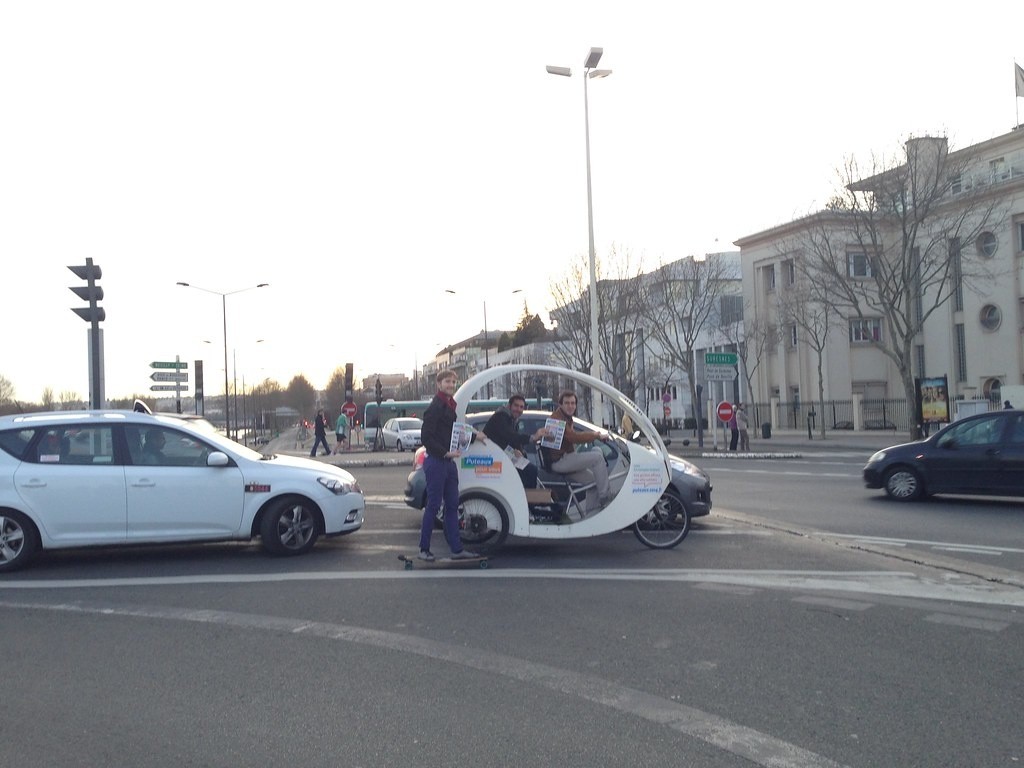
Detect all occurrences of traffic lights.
[304,420,309,428]
[67,266,105,323]
[375,380,384,403]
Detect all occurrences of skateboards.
[397,554,489,570]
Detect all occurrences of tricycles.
[436,363,690,558]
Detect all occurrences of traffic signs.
[150,362,189,393]
[703,354,740,382]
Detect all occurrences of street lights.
[546,47,613,428]
[444,289,524,400]
[175,282,267,448]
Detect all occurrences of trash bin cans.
[762,423,771,439]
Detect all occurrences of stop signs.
[663,408,671,415]
[341,401,357,417]
[717,402,734,421]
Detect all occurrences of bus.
[364,398,555,447]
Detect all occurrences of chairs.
[535,439,582,486]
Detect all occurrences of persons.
[310,409,331,457]
[622,411,634,441]
[416,370,487,561]
[730,405,739,450]
[482,395,550,491]
[548,391,621,514]
[1003,400,1013,409]
[736,402,751,452]
[142,429,167,464]
[920,386,946,402]
[333,410,352,455]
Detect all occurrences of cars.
[248,436,269,446]
[378,416,426,452]
[833,420,896,430]
[862,410,1024,502]
[0,400,367,573]
[403,411,713,531]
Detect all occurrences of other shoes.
[416,549,435,562]
[450,549,479,559]
[530,509,554,522]
[599,489,620,508]
